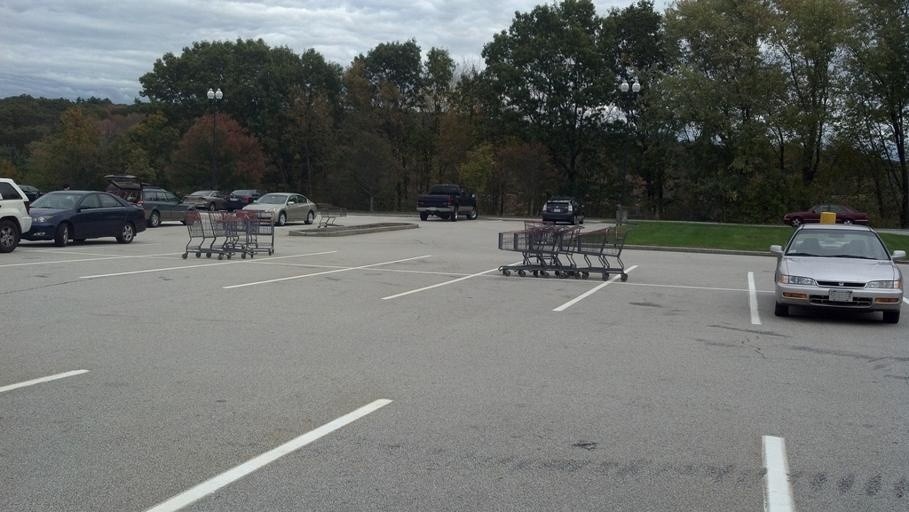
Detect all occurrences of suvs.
[543,199,587,227]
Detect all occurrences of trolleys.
[496,218,635,283]
[172,201,277,262]
[318,202,348,228]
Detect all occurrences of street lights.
[207,86,225,190]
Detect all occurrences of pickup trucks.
[413,180,480,222]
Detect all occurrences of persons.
[58,184,70,209]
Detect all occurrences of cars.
[770,222,906,323]
[780,200,875,228]
